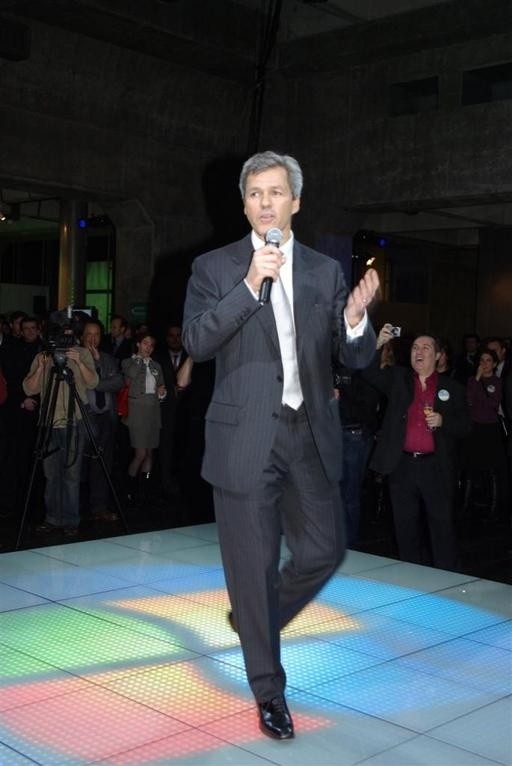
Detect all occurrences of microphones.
[258,227,284,304]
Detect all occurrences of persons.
[182,151,381,742]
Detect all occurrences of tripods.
[12,365,132,551]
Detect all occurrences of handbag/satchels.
[114,376,131,417]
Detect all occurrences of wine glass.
[422,398,438,433]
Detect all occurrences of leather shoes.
[259,695,293,739]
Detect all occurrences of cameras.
[386,326,402,338]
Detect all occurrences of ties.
[269,276,303,411]
